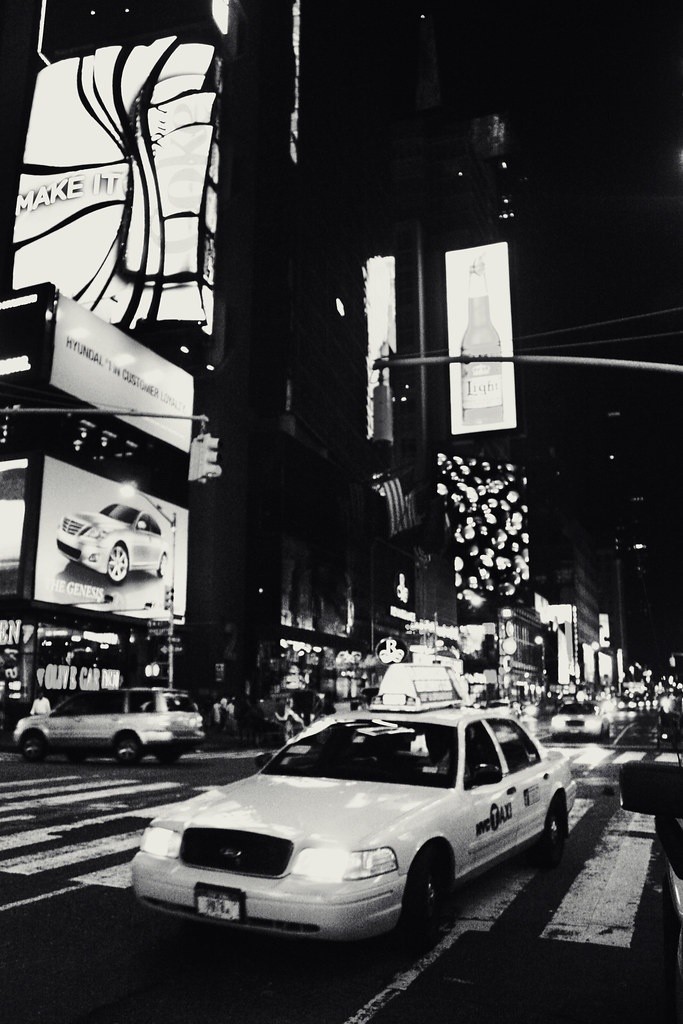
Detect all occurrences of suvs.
[12,687,205,767]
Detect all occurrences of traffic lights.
[164,589,170,610]
[188,433,223,482]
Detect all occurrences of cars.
[132,663,578,957]
[549,691,610,743]
[55,502,169,586]
